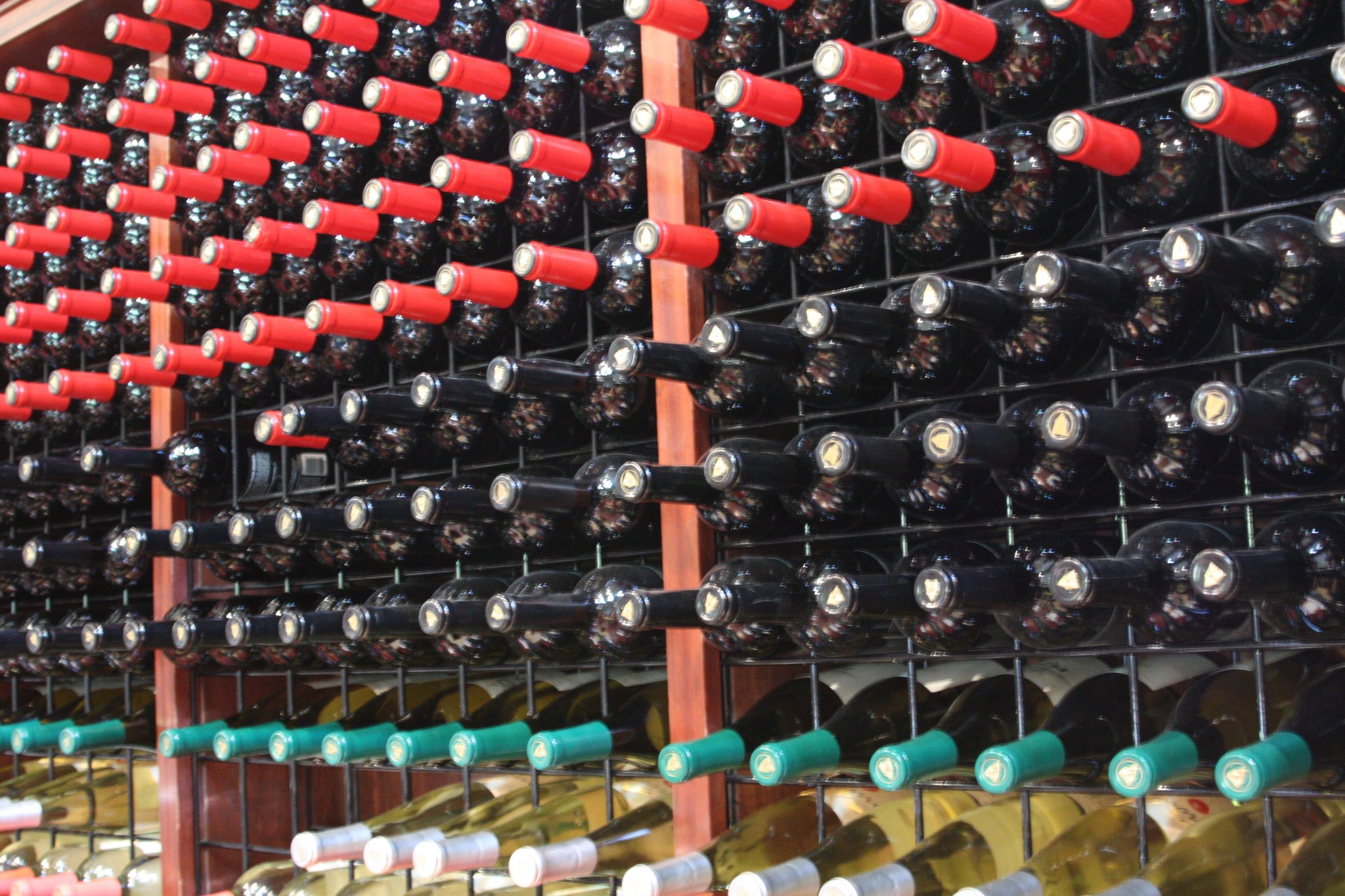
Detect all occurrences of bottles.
[0,0,1345,896]
